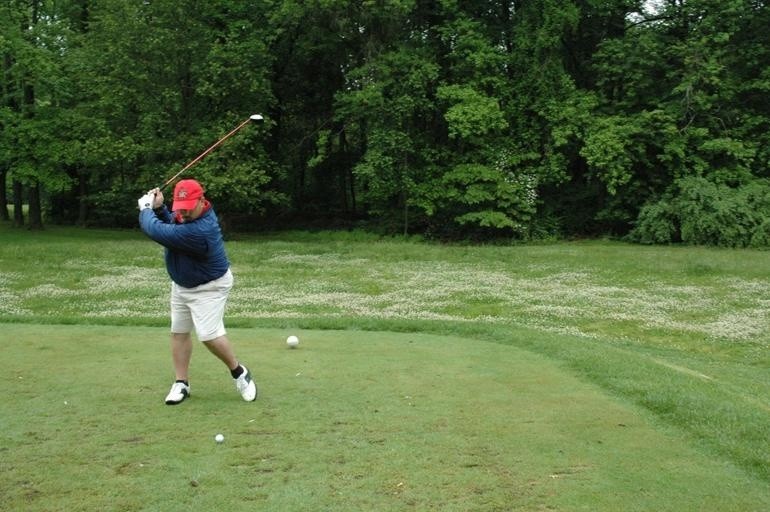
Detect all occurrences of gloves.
[139,193,154,210]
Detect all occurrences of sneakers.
[165,381,190,404]
[232,364,257,401]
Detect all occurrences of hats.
[171,178,204,211]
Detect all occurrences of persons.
[138,178,257,406]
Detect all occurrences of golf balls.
[215,434,225,444]
[287,336,298,348]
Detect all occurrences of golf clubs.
[134,114,263,212]
